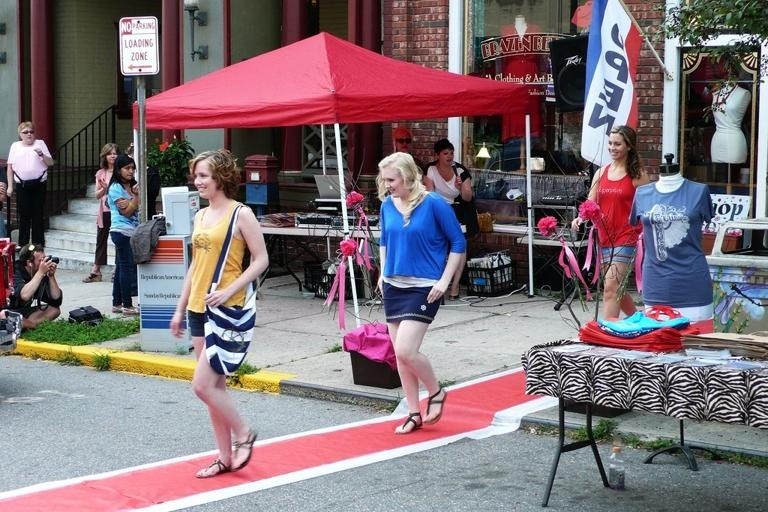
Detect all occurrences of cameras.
[43,255,60,265]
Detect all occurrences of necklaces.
[716,84,738,105]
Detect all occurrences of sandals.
[194,460,231,479]
[231,429,257,472]
[423,388,448,428]
[393,411,423,439]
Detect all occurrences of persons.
[710,80,751,164]
[1,243,62,332]
[0,182,7,238]
[7,122,55,246]
[571,125,649,319]
[423,139,479,301]
[106,154,141,315]
[131,155,161,297]
[629,171,714,323]
[377,151,467,434]
[82,144,120,283]
[393,126,423,176]
[501,15,541,143]
[171,147,270,478]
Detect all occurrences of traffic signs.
[118,15,159,76]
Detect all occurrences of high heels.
[82,272,103,284]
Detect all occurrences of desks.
[521,344,768,507]
[257,225,469,306]
[516,236,592,310]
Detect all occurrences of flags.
[581,0,645,167]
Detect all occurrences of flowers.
[540,215,558,236]
[578,200,601,230]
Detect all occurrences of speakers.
[548,35,592,112]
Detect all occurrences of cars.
[478,144,546,196]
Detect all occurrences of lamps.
[185,0,207,60]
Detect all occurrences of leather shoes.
[448,284,460,301]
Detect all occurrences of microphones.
[449,161,459,177]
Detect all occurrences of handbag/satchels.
[12,167,48,189]
[204,290,261,380]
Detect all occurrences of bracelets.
[39,153,43,156]
[128,202,138,210]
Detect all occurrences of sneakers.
[109,305,142,316]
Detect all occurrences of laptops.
[313,174,340,198]
